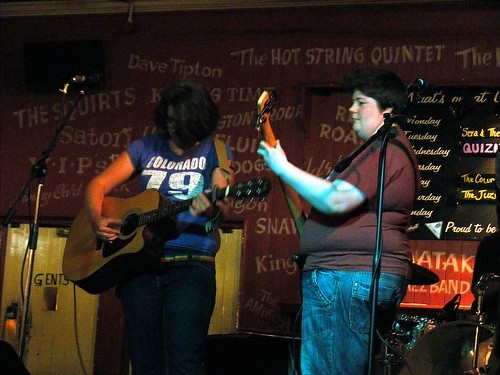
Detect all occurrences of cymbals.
[402,320,500,375]
[407,260,440,286]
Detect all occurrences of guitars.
[253,89,308,299]
[62,176,271,295]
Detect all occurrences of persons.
[83,79,237,375]
[256,65,421,375]
[470,194,500,374]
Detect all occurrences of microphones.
[71,69,103,84]
[406,79,424,95]
